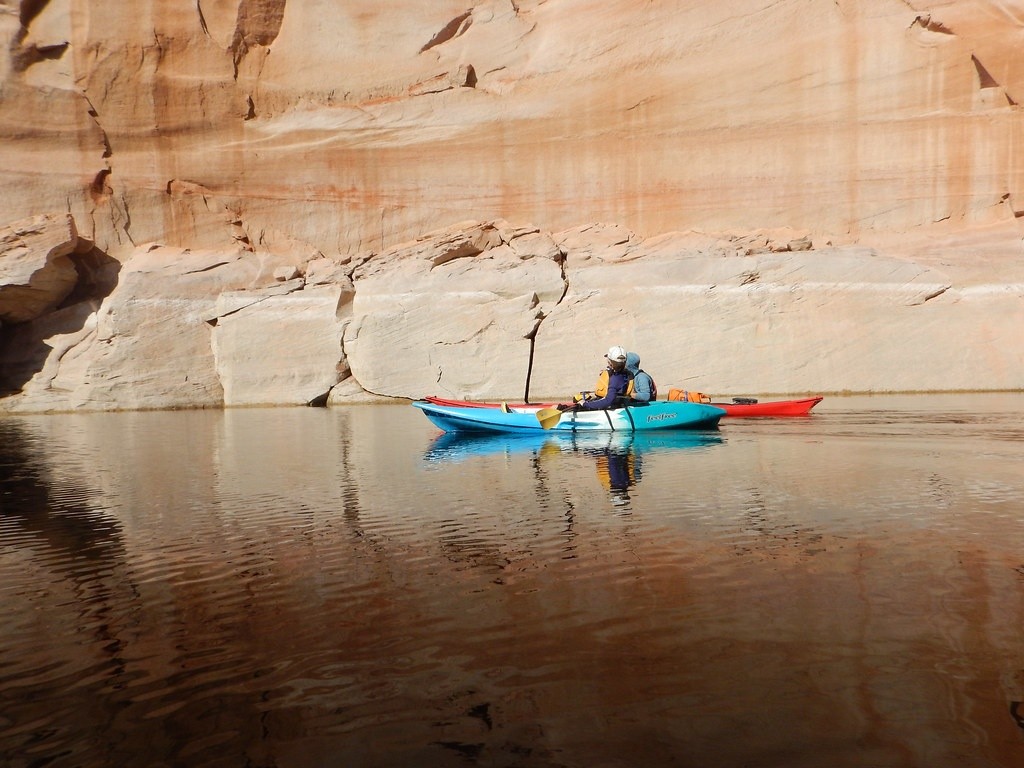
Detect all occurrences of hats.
[603,346,627,363]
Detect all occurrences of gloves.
[575,399,586,407]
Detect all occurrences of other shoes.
[501,401,513,413]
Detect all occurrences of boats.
[412,401,727,435]
[425,395,825,416]
[424,431,728,462]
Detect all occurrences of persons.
[500,347,634,413]
[625,352,657,402]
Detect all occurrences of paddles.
[535,405,577,429]
